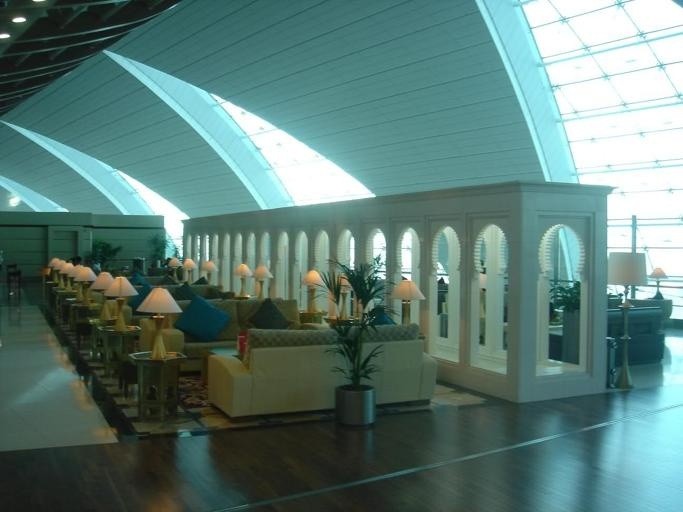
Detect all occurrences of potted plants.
[548,280,580,364]
[316,252,400,426]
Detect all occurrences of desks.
[629,298,673,318]
[67,299,139,361]
[98,326,186,423]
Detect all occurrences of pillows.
[176,298,230,342]
[249,300,292,330]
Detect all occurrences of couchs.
[548,305,665,366]
[207,324,438,419]
[141,298,324,371]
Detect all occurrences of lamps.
[252,265,274,297]
[395,279,424,326]
[303,270,326,313]
[235,264,253,299]
[135,286,182,358]
[649,268,669,300]
[606,252,648,390]
[104,276,138,331]
[44,256,246,306]
